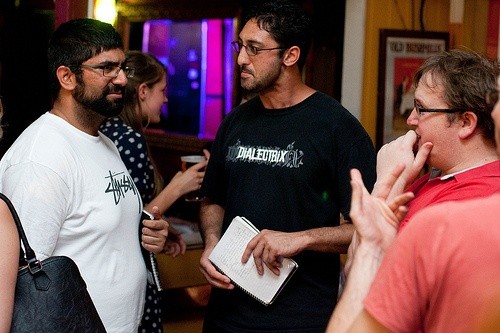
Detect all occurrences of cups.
[182,155,205,201]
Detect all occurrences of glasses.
[231,40,285,55]
[69,64,135,79]
[415,101,467,116]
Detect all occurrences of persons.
[325,45,500,289]
[99,50,210,333]
[325,74,500,333]
[198,0,377,333]
[0,18,170,333]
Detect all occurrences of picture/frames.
[376,28,449,172]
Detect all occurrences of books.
[207,216,299,306]
[138,209,163,293]
[168,223,204,250]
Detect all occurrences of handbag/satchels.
[0,193,106,333]
